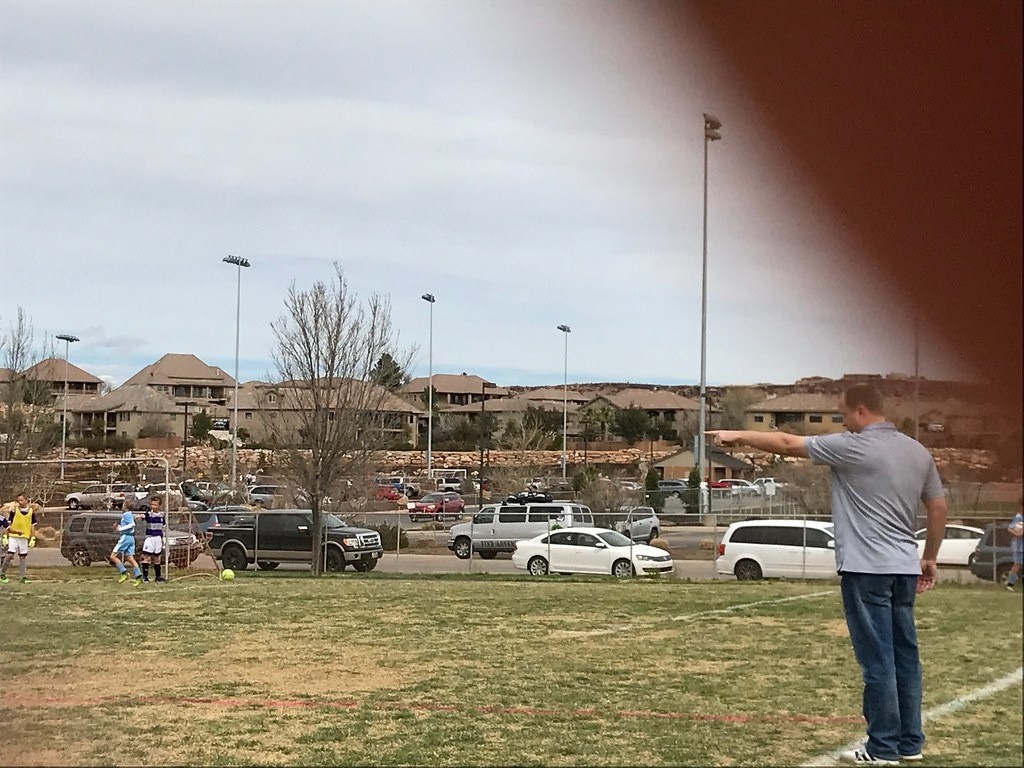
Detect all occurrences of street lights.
[698,112,721,516]
[558,325,571,481]
[55,334,79,478]
[422,293,436,480]
[224,255,251,491]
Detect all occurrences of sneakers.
[0,573,9,583]
[19,577,31,583]
[902,753,923,759]
[846,747,900,765]
[133,578,142,587]
[119,571,130,583]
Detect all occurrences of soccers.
[221,569,235,581]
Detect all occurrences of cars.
[719,477,788,496]
[60,512,200,568]
[913,524,985,569]
[619,482,642,490]
[65,483,135,512]
[136,479,308,509]
[716,521,841,582]
[657,480,687,499]
[606,507,661,545]
[408,490,464,522]
[370,475,546,500]
[970,523,1024,585]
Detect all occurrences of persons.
[246,472,256,485]
[110,499,143,587]
[1005,513,1024,591]
[0,492,37,584]
[702,385,948,765]
[135,496,166,583]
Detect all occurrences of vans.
[447,492,594,560]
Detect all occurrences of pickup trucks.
[202,509,383,574]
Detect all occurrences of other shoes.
[155,576,166,583]
[143,577,150,584]
[1005,580,1013,591]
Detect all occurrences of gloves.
[2,535,7,546]
[28,536,35,548]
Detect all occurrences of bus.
[511,527,675,579]
